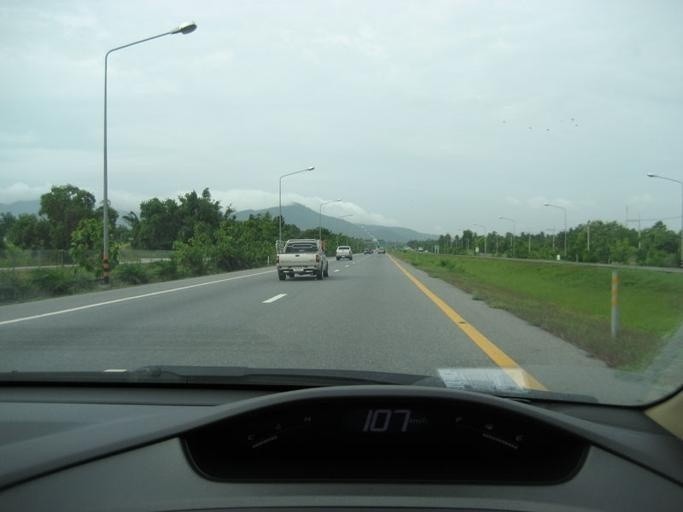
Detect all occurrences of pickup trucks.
[277,238,328,280]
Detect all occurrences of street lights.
[544,203,567,256]
[647,173,683,185]
[278,166,314,242]
[320,198,354,238]
[498,216,516,257]
[103,20,197,259]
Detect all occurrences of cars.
[364,248,385,254]
[336,246,352,260]
